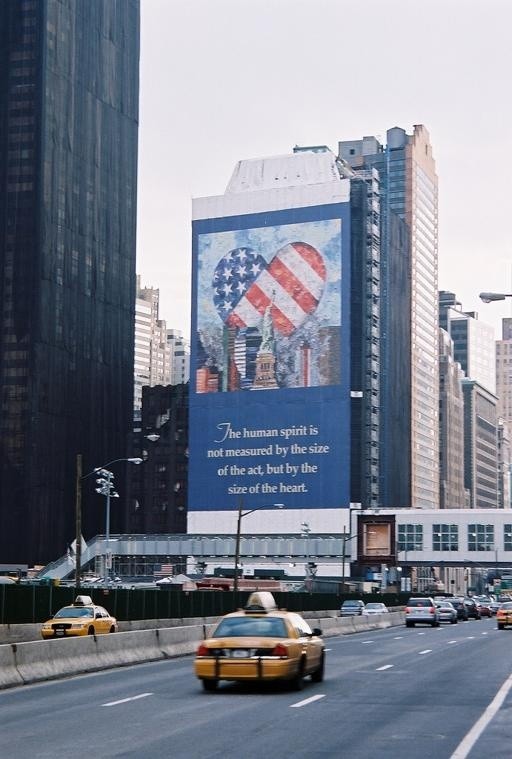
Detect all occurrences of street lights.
[233,493,285,591]
[71,450,145,606]
[122,433,161,535]
[300,518,313,583]
[394,544,421,592]
[93,464,120,590]
[342,524,378,591]
[475,289,512,304]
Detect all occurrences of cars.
[361,602,389,617]
[496,602,512,630]
[39,595,120,638]
[432,593,512,621]
[191,589,328,691]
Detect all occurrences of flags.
[154,565,173,578]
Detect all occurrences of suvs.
[405,596,440,628]
[339,598,365,617]
